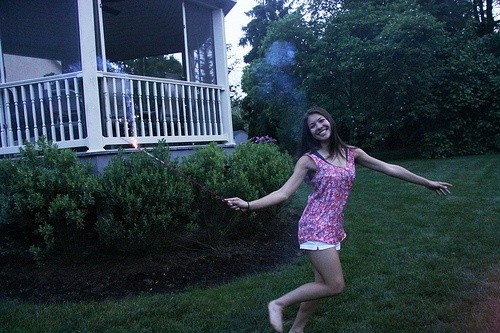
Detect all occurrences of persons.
[222,107,452,333]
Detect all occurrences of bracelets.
[246,201,250,212]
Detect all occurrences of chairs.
[45,89,85,139]
